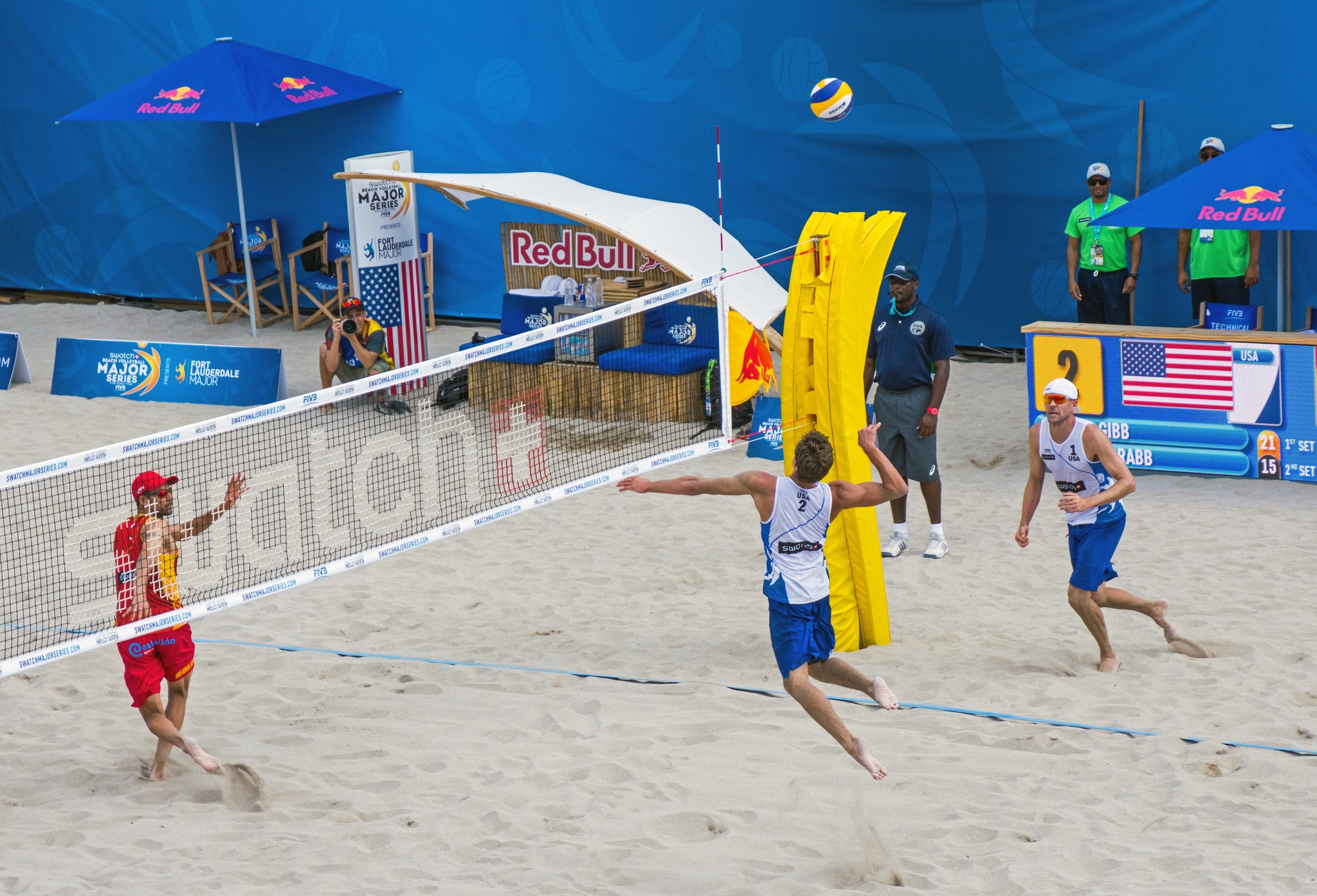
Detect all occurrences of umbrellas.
[1084,122,1317,332]
[56,37,405,340]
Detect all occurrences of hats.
[885,261,919,280]
[1199,137,1225,153]
[132,471,178,502]
[341,297,364,315]
[1087,162,1110,179]
[1044,378,1080,412]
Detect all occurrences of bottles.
[585,279,595,307]
[564,277,573,306]
[594,277,604,305]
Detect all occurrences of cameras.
[471,331,485,345]
[334,318,357,335]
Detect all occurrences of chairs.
[345,233,436,334]
[1292,305,1317,334]
[287,221,350,333]
[1186,301,1263,331]
[459,292,564,415]
[599,301,718,422]
[196,219,291,327]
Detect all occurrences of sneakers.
[924,532,948,559]
[881,532,909,557]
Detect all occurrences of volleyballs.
[811,77,854,121]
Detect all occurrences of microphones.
[887,289,890,295]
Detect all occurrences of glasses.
[342,299,361,309]
[148,488,172,498]
[1200,153,1219,159]
[1043,394,1069,404]
[1088,178,1108,186]
[888,277,911,285]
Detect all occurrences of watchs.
[1128,272,1140,280]
[924,406,943,417]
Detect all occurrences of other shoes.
[373,403,392,415]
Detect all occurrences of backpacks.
[436,368,468,409]
[700,364,754,430]
[301,227,329,272]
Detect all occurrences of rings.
[132,608,137,613]
[236,487,240,491]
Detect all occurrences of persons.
[1177,137,1261,319]
[1015,378,1176,673]
[1064,162,1145,326]
[319,298,395,417]
[864,261,956,559]
[615,422,910,782]
[114,472,251,782]
